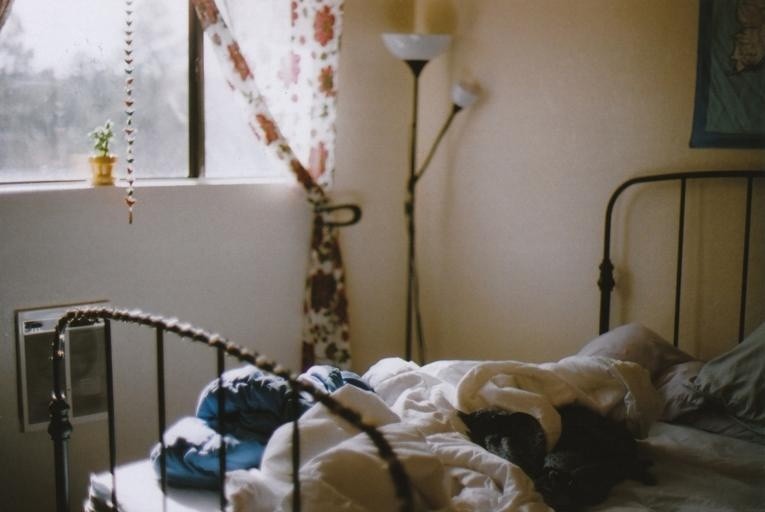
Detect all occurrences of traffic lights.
[681,319,765,426]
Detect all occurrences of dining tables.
[85,119,118,187]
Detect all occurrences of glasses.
[379,34,476,367]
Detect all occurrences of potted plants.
[688,0,765,148]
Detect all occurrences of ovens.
[48,169,765,511]
